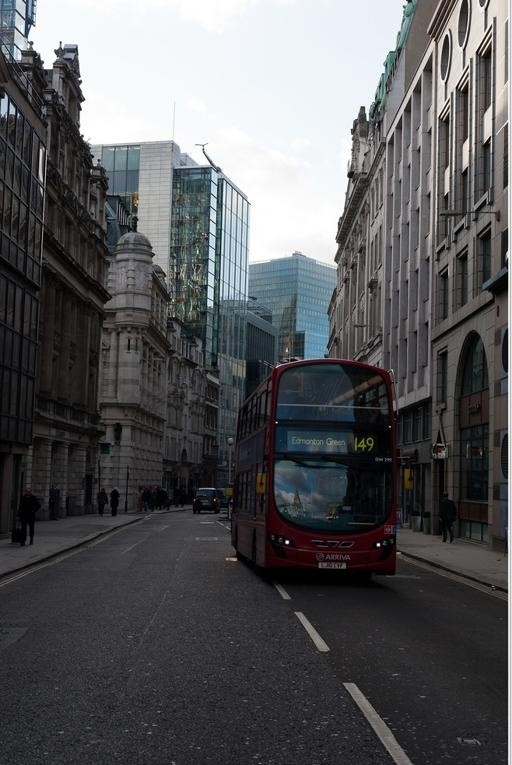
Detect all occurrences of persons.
[438,492,457,544]
[109,486,121,517]
[140,485,186,513]
[96,487,109,518]
[16,485,41,547]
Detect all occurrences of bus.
[231,358,397,580]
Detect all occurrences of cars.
[193,487,230,514]
